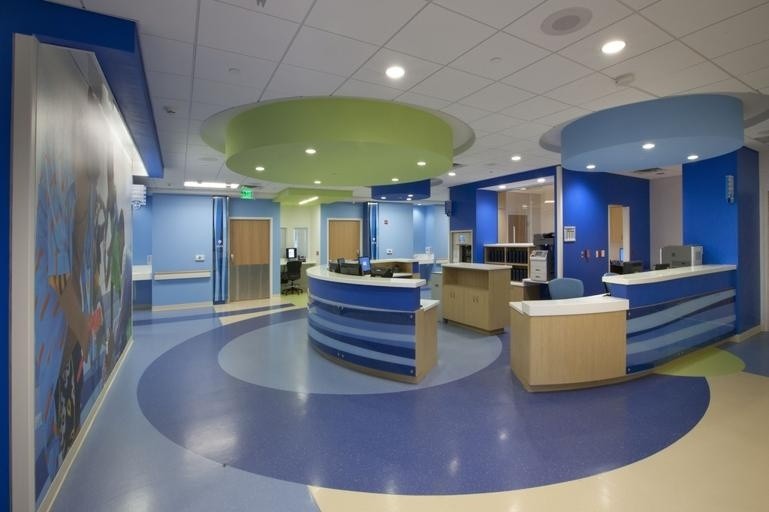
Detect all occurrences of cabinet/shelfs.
[484,247,530,283]
[441,262,512,335]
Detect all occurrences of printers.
[530,232,556,282]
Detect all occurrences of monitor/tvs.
[286,248,297,258]
[623,262,642,274]
[339,263,361,275]
[337,258,345,264]
[358,256,371,272]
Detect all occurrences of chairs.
[281,261,303,296]
[548,278,584,300]
